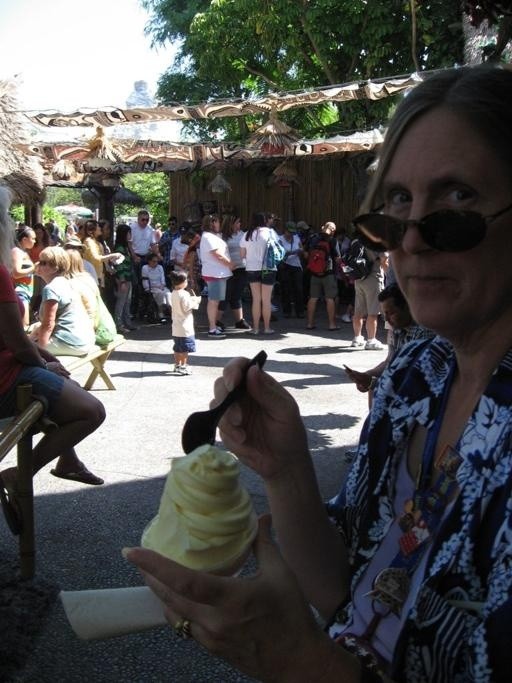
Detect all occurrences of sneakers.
[351,335,364,347]
[174,365,192,375]
[341,314,351,322]
[208,319,274,338]
[365,339,387,349]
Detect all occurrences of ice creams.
[142,443,256,572]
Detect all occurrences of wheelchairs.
[136,260,174,324]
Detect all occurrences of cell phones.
[344,363,352,374]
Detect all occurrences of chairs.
[133,276,157,322]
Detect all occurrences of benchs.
[55,332,125,391]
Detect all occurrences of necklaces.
[360,355,495,623]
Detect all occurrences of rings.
[174,617,190,642]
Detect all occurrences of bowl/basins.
[137,510,260,579]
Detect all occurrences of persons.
[1,186,105,538]
[11,208,433,409]
[120,64,512,683]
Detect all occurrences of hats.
[286,221,312,233]
[62,235,87,254]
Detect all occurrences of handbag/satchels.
[266,239,286,268]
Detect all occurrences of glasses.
[142,219,149,222]
[169,223,175,225]
[351,202,511,253]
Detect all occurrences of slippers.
[51,463,104,485]
[306,326,316,329]
[0,481,21,536]
[329,325,342,330]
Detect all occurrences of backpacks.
[308,234,335,277]
[335,238,374,281]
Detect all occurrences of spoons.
[182,350,267,455]
